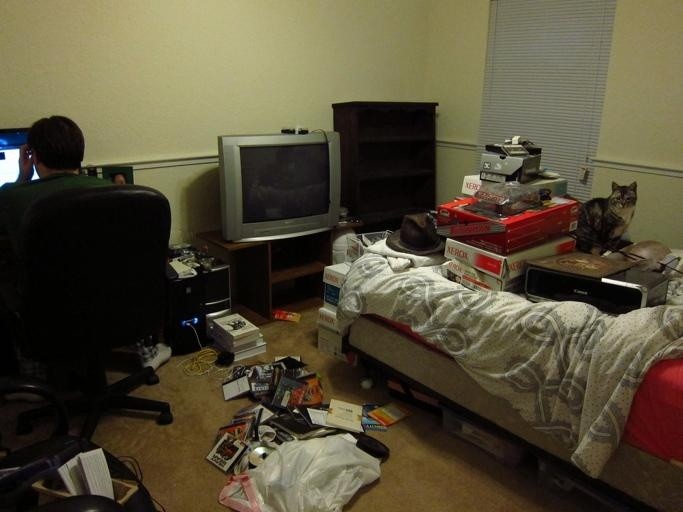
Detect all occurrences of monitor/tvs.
[217,130,341,243]
[0,128,40,187]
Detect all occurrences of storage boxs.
[314,264,349,364]
[436,194,579,294]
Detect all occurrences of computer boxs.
[182,245,231,345]
[160,253,206,356]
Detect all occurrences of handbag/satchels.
[233,424,280,474]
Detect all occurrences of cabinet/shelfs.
[332,106,436,230]
[205,230,333,325]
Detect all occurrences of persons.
[0,114,173,403]
[109,171,127,185]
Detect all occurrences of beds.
[338,206,681,509]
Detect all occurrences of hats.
[386,213,445,256]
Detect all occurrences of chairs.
[0,177,172,460]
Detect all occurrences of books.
[31,446,140,506]
[360,401,387,431]
[266,403,365,444]
[209,312,267,362]
[366,397,416,427]
[324,397,363,434]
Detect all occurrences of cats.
[578,179,638,257]
